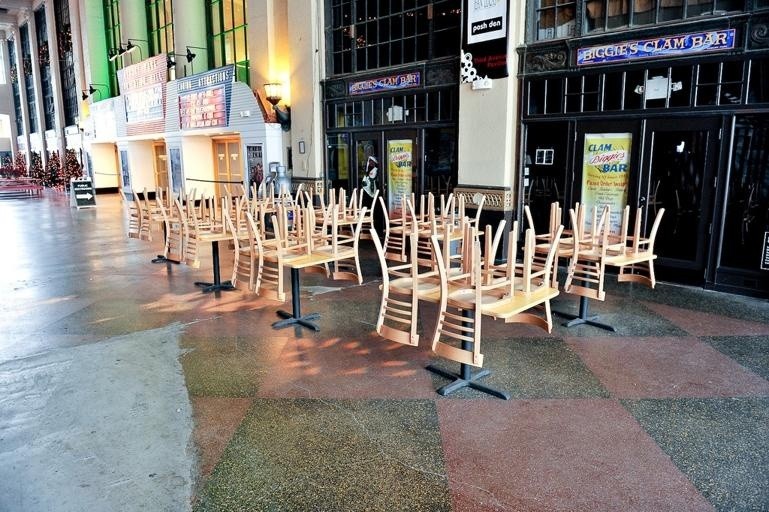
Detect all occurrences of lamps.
[127,39,147,52]
[82,89,102,100]
[166,53,189,68]
[119,43,142,62]
[186,46,206,63]
[108,48,123,69]
[264,83,292,131]
[89,84,110,95]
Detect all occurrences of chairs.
[222,203,366,331]
[370,221,566,400]
[155,194,266,294]
[300,187,379,268]
[119,187,197,265]
[224,181,302,233]
[379,192,486,267]
[524,201,666,333]
